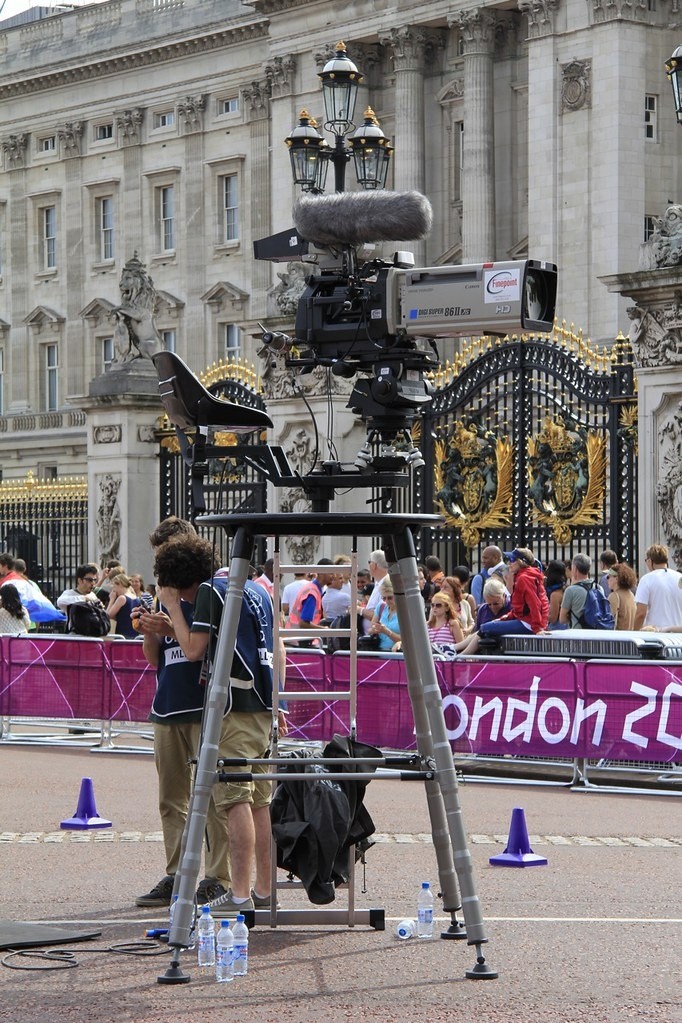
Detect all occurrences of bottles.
[232,915,248,975]
[170,895,185,952]
[215,920,235,981]
[417,882,433,938]
[198,906,215,967]
[186,906,196,949]
[396,920,415,939]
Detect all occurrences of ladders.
[239,533,386,930]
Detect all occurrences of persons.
[129,516,232,906]
[0,552,67,634]
[57,563,104,634]
[634,544,682,633]
[252,545,570,658]
[599,550,637,599]
[153,531,291,916]
[559,553,604,629]
[602,563,636,630]
[92,560,156,639]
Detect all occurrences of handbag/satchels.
[320,618,332,654]
[65,602,110,637]
[357,634,382,651]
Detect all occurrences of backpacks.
[571,581,615,629]
[462,573,485,601]
[326,613,365,654]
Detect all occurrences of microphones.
[290,189,433,248]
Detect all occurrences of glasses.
[368,560,377,564]
[430,603,443,609]
[607,576,616,580]
[486,598,501,606]
[505,555,519,562]
[643,557,653,563]
[381,595,393,601]
[81,577,98,582]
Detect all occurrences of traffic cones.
[60,777,112,830]
[489,808,548,868]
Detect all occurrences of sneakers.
[136,876,173,907]
[197,888,254,918]
[193,880,228,906]
[252,889,281,910]
[432,643,457,658]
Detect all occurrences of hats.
[502,550,535,566]
[602,569,619,576]
[358,583,374,596]
